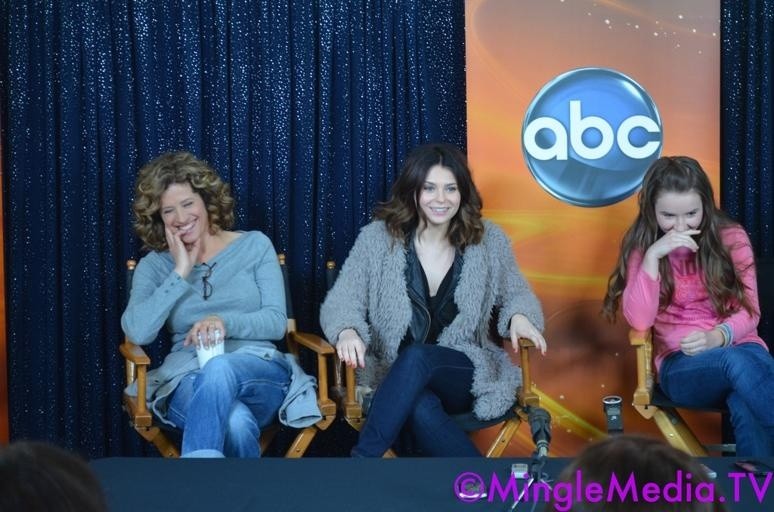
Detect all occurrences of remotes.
[512,464,528,479]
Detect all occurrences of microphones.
[528,409,551,461]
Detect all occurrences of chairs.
[119,253,540,458]
[629,328,736,457]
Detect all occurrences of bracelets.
[715,323,733,348]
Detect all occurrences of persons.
[319,145,547,459]
[599,157,774,457]
[543,434,731,512]
[0,442,105,512]
[121,151,292,458]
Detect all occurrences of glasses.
[199,261,216,300]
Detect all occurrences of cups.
[193,331,223,365]
